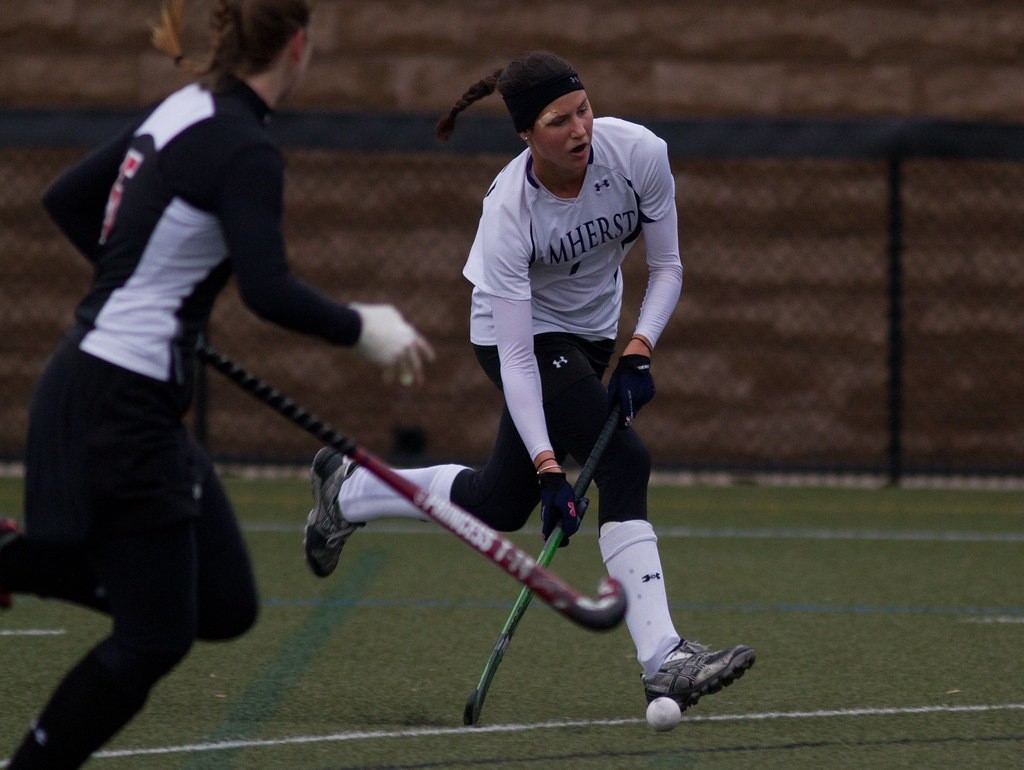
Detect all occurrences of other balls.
[646,697,682,732]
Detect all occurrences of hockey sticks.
[202,345,628,635]
[463,392,622,725]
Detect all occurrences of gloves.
[537,471,588,547]
[609,354,655,431]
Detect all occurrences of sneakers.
[640,638,755,713]
[303,445,366,578]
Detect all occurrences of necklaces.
[537,458,558,471]
[630,337,652,354]
[536,465,562,475]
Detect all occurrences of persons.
[305,51,757,710]
[1,2,436,770]
